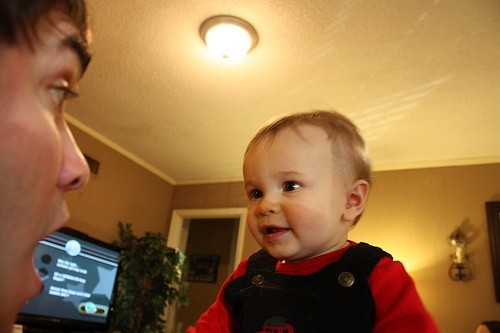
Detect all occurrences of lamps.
[200,15,258,62]
[446,231,474,282]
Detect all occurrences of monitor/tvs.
[14,226,125,333]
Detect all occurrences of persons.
[0,0,92,333]
[186,111,440,333]
